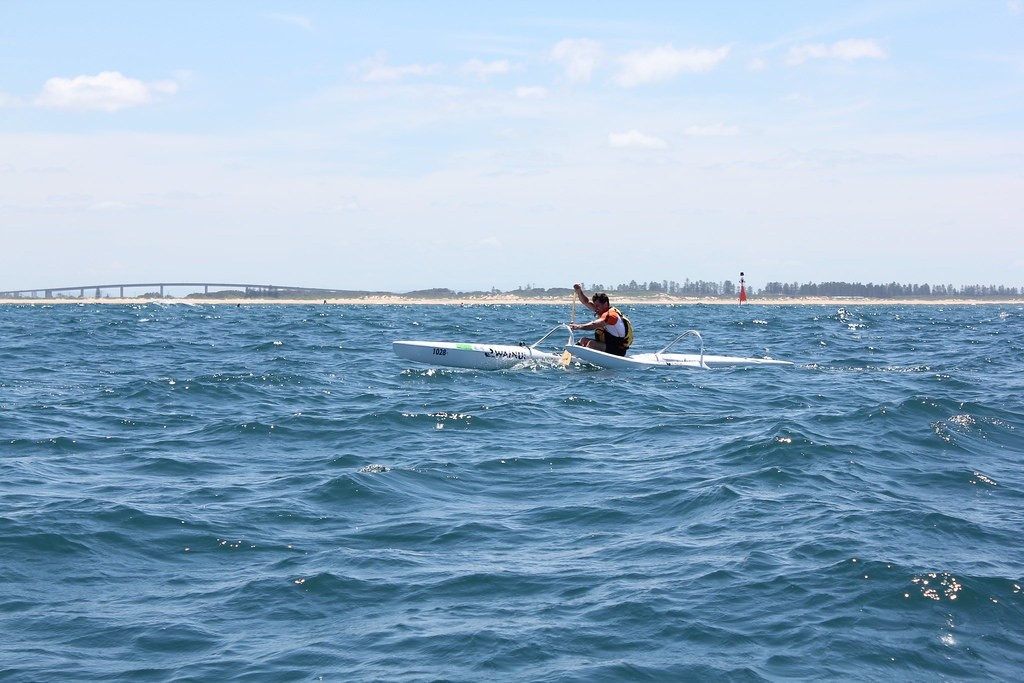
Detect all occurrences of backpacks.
[610,306,633,348]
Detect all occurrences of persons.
[568,282,629,357]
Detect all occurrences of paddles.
[560,283,580,365]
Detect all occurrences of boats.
[393,322,793,373]
